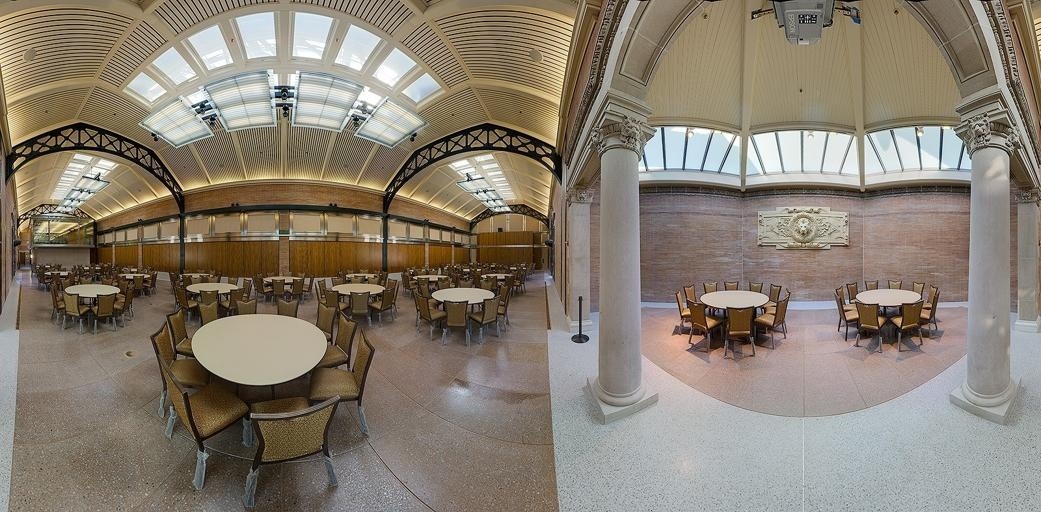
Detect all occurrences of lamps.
[52,206,79,215]
[199,68,278,133]
[290,68,364,134]
[486,199,491,203]
[475,190,480,195]
[482,189,489,194]
[190,100,213,116]
[58,197,87,210]
[469,190,503,201]
[276,102,293,118]
[410,133,417,142]
[455,176,492,193]
[86,189,91,194]
[149,132,159,142]
[354,96,430,149]
[465,173,473,182]
[135,94,216,151]
[93,172,101,181]
[274,85,295,102]
[76,199,81,203]
[65,188,96,201]
[69,198,76,202]
[73,175,111,194]
[201,112,217,128]
[355,99,377,117]
[489,206,513,213]
[498,206,503,208]
[480,200,506,208]
[78,188,85,194]
[492,199,498,202]
[351,113,367,131]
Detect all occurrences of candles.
[346,273,375,280]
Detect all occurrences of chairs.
[420,283,442,309]
[243,279,252,300]
[257,272,264,279]
[468,294,501,345]
[116,280,128,296]
[266,272,275,276]
[113,271,118,280]
[287,279,304,300]
[102,278,113,285]
[106,289,132,328]
[458,279,474,287]
[228,277,238,285]
[54,283,63,301]
[91,293,116,334]
[304,274,313,295]
[686,298,723,352]
[369,277,380,285]
[379,282,401,312]
[271,278,285,306]
[192,277,200,283]
[197,301,219,320]
[325,292,349,318]
[337,271,345,277]
[833,291,858,341]
[754,295,790,350]
[252,275,257,294]
[196,268,205,273]
[913,282,925,295]
[308,330,376,437]
[49,285,66,325]
[386,278,397,288]
[165,309,193,358]
[360,264,369,272]
[919,289,940,337]
[183,267,191,273]
[724,281,739,290]
[683,284,696,307]
[499,283,508,303]
[762,284,782,314]
[154,355,249,490]
[208,276,217,283]
[887,279,902,309]
[675,290,692,336]
[516,274,527,293]
[379,271,389,281]
[405,275,415,292]
[175,287,198,321]
[412,287,435,327]
[174,281,181,287]
[480,280,492,290]
[37,274,52,288]
[486,275,497,286]
[80,276,92,284]
[149,321,208,418]
[922,284,938,310]
[703,282,719,313]
[350,277,362,283]
[35,261,160,279]
[854,302,890,353]
[724,305,756,357]
[220,288,244,315]
[400,274,404,292]
[482,292,510,334]
[243,395,341,510]
[236,299,257,311]
[256,278,273,303]
[766,288,791,333]
[345,268,354,273]
[315,311,358,370]
[133,276,144,296]
[865,280,878,290]
[209,269,215,274]
[368,287,394,321]
[277,299,300,318]
[835,286,855,311]
[438,280,450,289]
[144,274,158,297]
[62,290,91,334]
[349,291,370,316]
[315,283,326,309]
[117,296,125,302]
[199,290,219,303]
[63,278,72,289]
[417,296,447,339]
[169,270,178,292]
[889,300,925,352]
[441,299,471,347]
[846,282,858,303]
[315,302,337,343]
[183,276,192,286]
[498,276,513,296]
[512,274,523,294]
[355,275,366,281]
[331,278,343,286]
[319,278,345,302]
[214,274,221,279]
[283,271,292,276]
[298,273,305,279]
[749,281,763,293]
